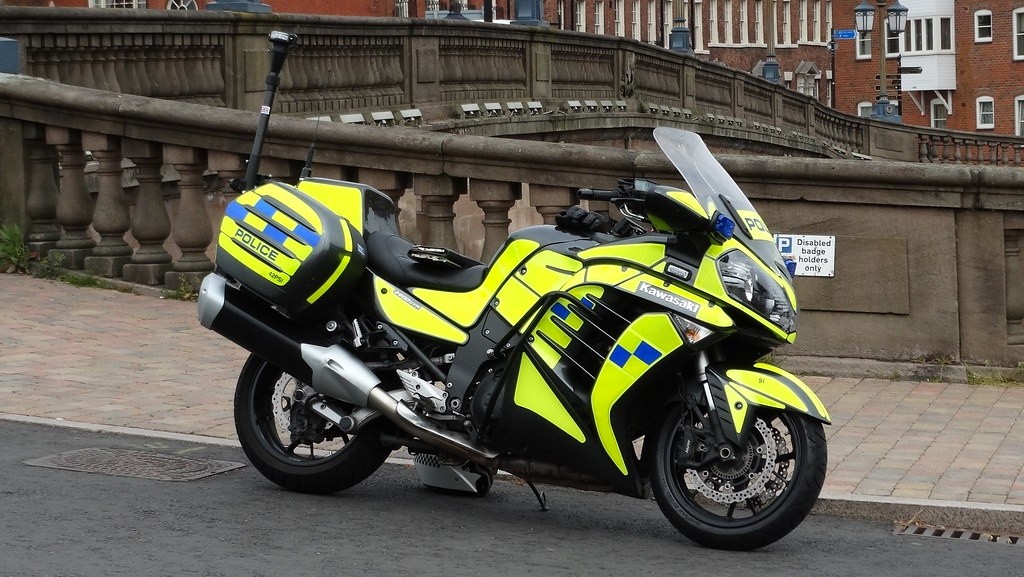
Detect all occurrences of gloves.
[554,205,607,236]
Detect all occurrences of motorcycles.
[197,31,831,553]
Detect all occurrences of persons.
[442,1,472,21]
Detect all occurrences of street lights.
[855,0,908,100]
